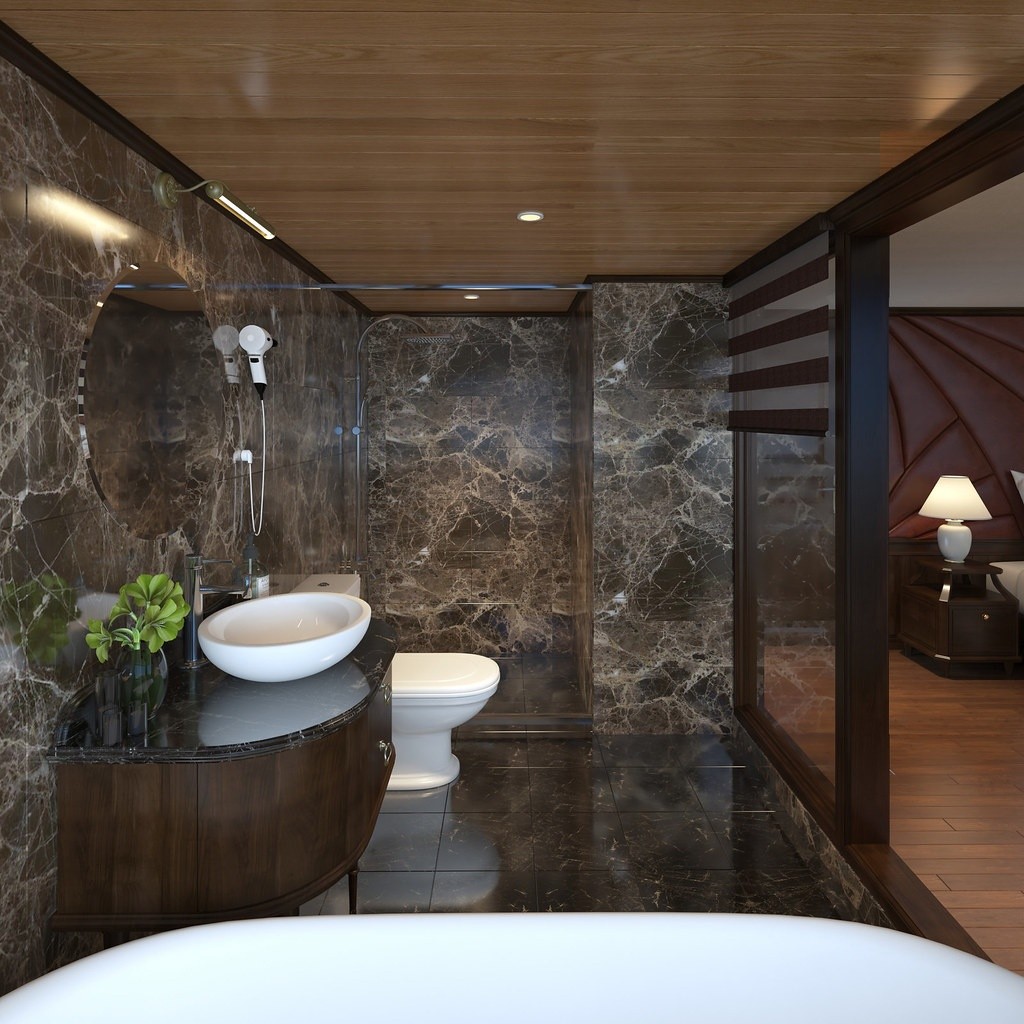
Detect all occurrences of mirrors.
[78,260,224,540]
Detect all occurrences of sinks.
[59,591,123,665]
[193,661,370,748]
[197,591,372,683]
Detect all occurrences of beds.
[986,561,1024,614]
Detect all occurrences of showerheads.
[362,381,378,403]
[399,334,450,345]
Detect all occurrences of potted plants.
[85,571,192,721]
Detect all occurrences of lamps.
[918,474,992,563]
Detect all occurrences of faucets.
[173,554,253,671]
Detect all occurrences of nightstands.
[897,561,1019,678]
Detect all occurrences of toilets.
[289,573,501,791]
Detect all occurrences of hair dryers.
[211,325,241,384]
[239,324,278,401]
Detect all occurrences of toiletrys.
[235,531,270,596]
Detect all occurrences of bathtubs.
[0,909,1020,1024]
